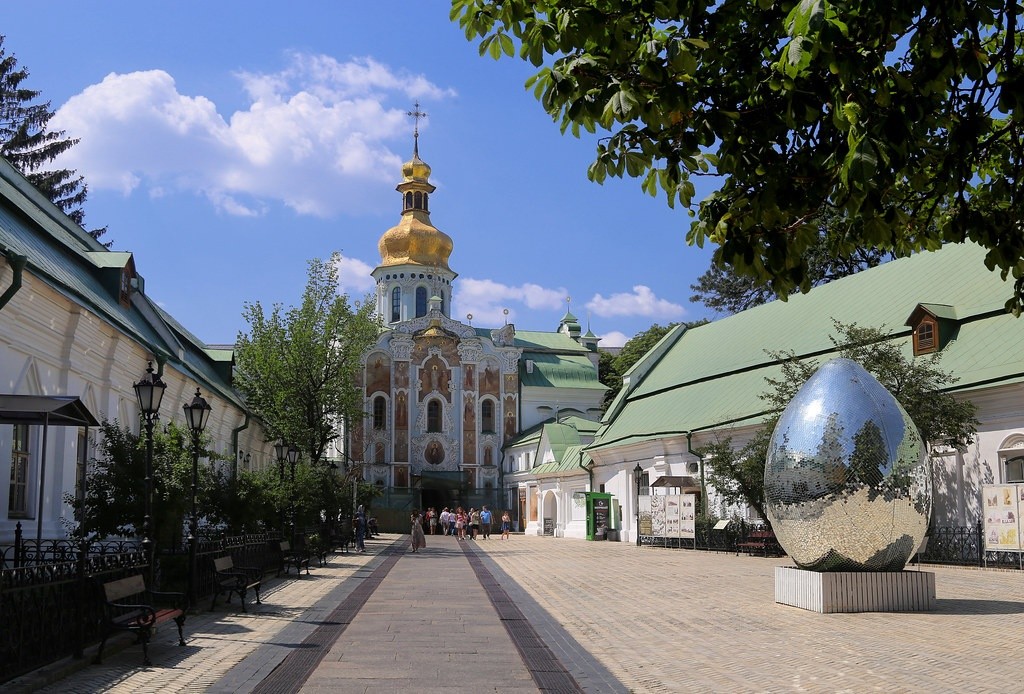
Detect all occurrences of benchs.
[736,532,781,557]
[211,557,262,613]
[277,527,357,579]
[96,576,187,666]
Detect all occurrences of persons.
[501,511,510,540]
[439,507,480,540]
[367,518,379,536]
[480,506,494,540]
[425,507,438,535]
[409,509,426,553]
[353,505,367,551]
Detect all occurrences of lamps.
[244,453,252,464]
[239,450,244,459]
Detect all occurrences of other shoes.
[459,537,462,540]
[411,549,419,553]
[355,547,359,551]
[361,549,366,552]
